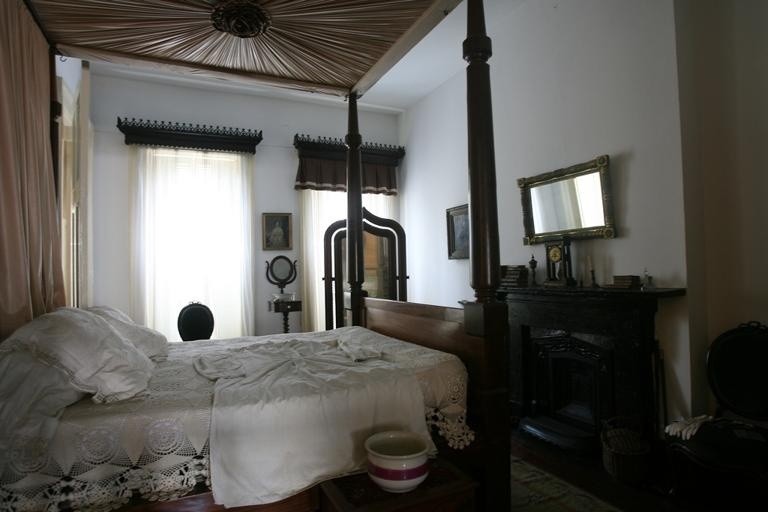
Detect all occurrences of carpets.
[509,453,622,512]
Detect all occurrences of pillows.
[3,305,152,403]
[84,303,170,365]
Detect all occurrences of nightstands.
[314,458,484,512]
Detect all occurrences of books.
[602,276,640,291]
[501,265,528,288]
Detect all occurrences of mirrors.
[515,153,619,247]
[265,255,297,295]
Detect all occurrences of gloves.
[678,414,714,440]
[665,416,685,436]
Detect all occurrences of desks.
[494,282,689,474]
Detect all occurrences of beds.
[0,1,513,512]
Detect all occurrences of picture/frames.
[261,212,293,252]
[445,204,470,262]
